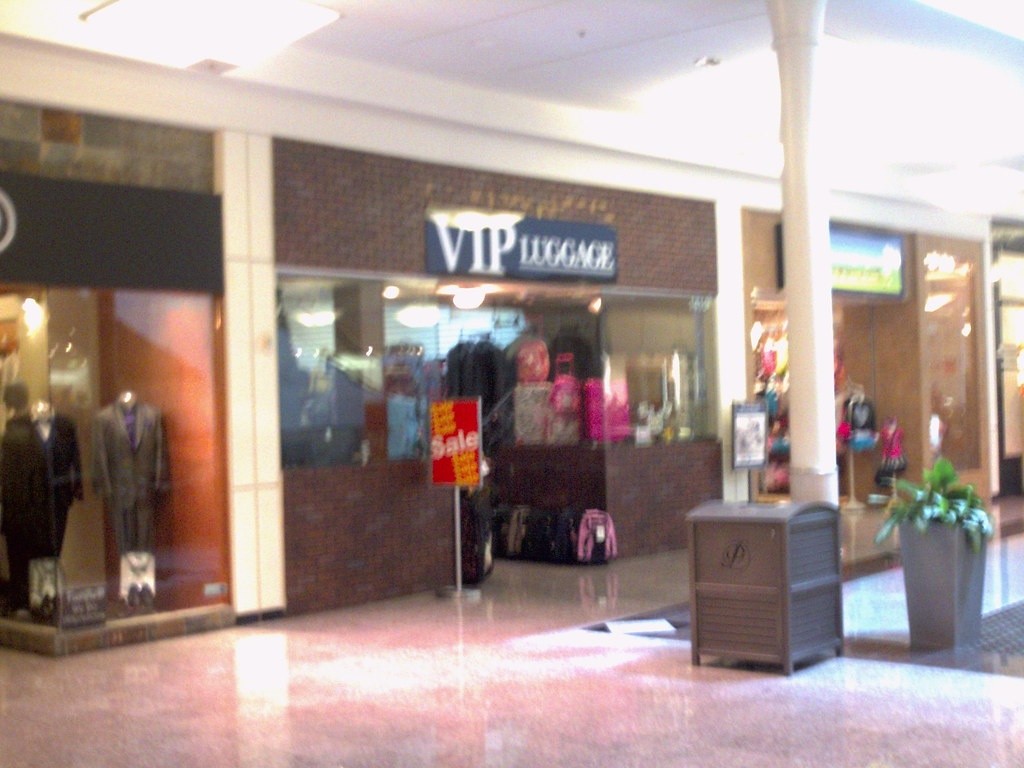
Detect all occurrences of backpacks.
[529,504,572,565]
[547,325,594,383]
[574,508,619,566]
[516,334,549,381]
[493,504,537,561]
[459,490,495,585]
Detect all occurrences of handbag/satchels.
[875,471,893,487]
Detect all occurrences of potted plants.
[868,454,996,651]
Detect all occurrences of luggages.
[544,353,583,448]
[582,375,636,450]
[513,384,559,451]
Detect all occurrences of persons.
[0,381,35,606]
[91,391,170,554]
[879,415,906,473]
[0,399,84,555]
[843,384,878,450]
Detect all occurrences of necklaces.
[123,551,151,577]
